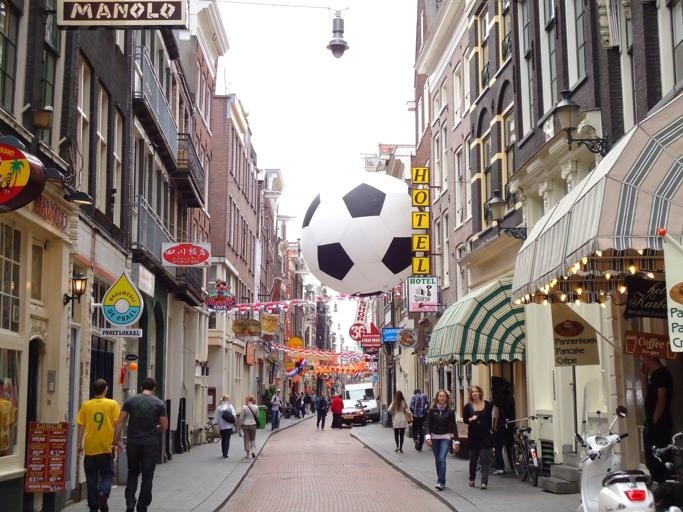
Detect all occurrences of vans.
[343,383,380,422]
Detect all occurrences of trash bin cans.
[258,405,267,428]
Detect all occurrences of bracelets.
[112,441,118,446]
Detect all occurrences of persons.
[464,384,493,489]
[639,354,674,507]
[388,391,407,453]
[111,377,168,511]
[269,388,343,431]
[76,379,121,511]
[215,394,236,457]
[239,395,259,458]
[0,381,15,454]
[408,389,430,450]
[492,378,515,475]
[426,390,461,490]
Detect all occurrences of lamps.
[63,273,88,306]
[0,134,93,205]
[488,190,526,240]
[555,90,608,158]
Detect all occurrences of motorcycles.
[649,432,683,512]
[573,406,655,512]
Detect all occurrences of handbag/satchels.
[403,399,412,424]
[221,403,235,424]
[254,415,261,428]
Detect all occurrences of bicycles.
[283,402,297,418]
[206,422,221,444]
[205,416,215,443]
[502,414,550,487]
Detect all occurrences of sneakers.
[126,504,135,512]
[481,483,487,489]
[415,440,419,450]
[98,492,108,512]
[435,483,443,490]
[469,482,475,487]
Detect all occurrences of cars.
[340,399,367,427]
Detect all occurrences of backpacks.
[412,393,427,418]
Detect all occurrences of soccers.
[300,170,427,297]
